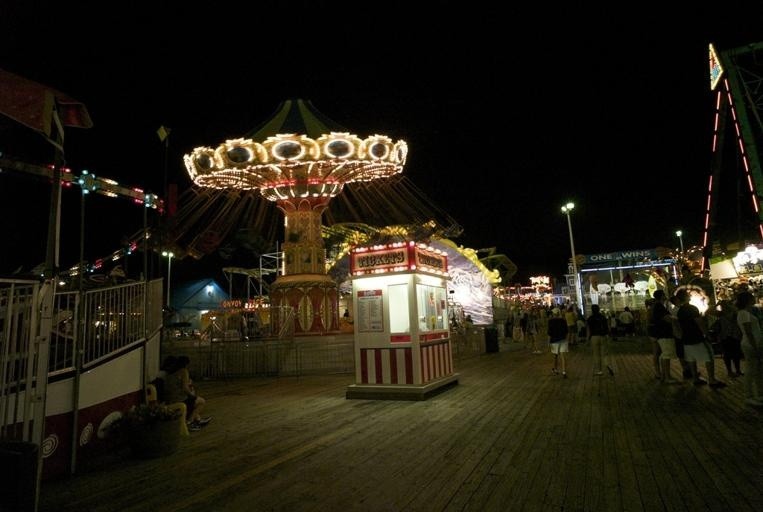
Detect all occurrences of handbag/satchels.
[672,322,683,339]
[710,319,721,335]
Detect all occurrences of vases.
[132,425,181,459]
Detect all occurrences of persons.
[162,355,212,431]
[344,309,349,317]
[504,278,763,408]
[466,315,473,324]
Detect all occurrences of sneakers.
[550,369,568,378]
[595,365,615,376]
[745,395,761,407]
[694,377,727,388]
[728,370,745,377]
[188,416,212,431]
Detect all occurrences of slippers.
[654,373,677,384]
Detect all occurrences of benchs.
[146,381,190,441]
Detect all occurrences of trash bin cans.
[484,326,499,354]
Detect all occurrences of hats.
[550,308,560,315]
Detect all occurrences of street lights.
[560,200,583,314]
[162,249,176,306]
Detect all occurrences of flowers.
[115,397,182,424]
